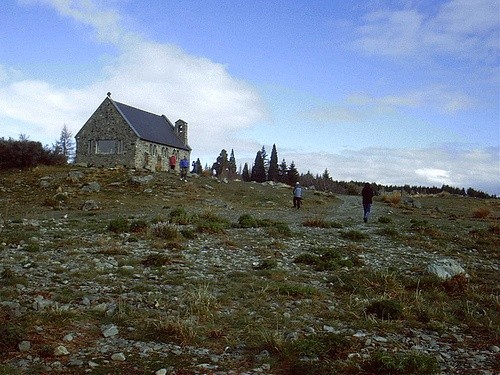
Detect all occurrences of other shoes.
[363,217,368,223]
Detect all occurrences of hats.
[296,182,299,184]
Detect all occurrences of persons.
[192,161,197,173]
[361,183,373,223]
[293,181,302,209]
[180,156,190,182]
[169,153,176,169]
